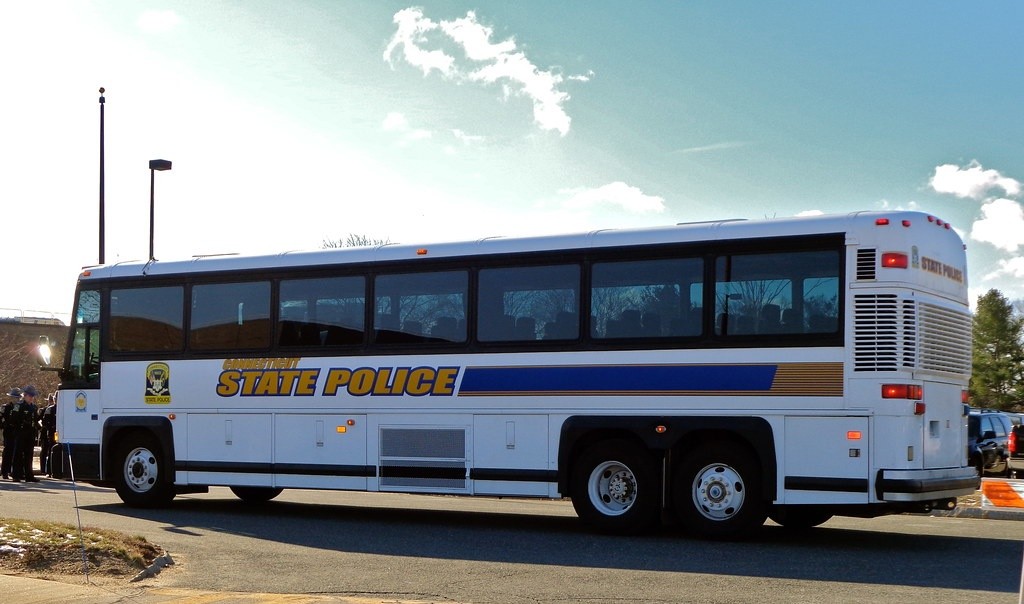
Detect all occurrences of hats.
[44,393,53,400]
[23,385,38,395]
[6,387,24,397]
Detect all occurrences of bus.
[40,208,983,541]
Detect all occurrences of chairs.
[280,302,840,346]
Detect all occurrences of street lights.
[149,158,172,262]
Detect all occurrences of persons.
[0,385,61,482]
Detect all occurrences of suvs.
[967,406,1024,471]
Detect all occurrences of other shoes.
[40,471,51,477]
[2,472,9,479]
[13,477,21,482]
[25,475,40,482]
